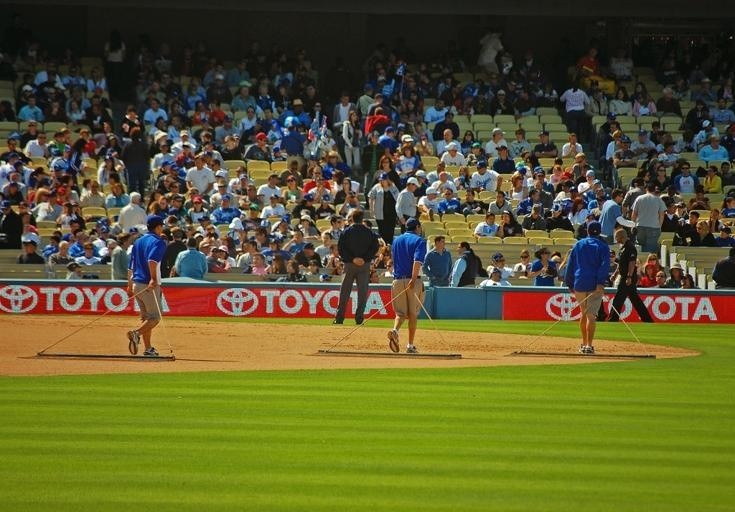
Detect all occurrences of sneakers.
[143,346,159,356]
[579,344,594,354]
[388,328,402,353]
[332,319,344,325]
[125,329,142,355]
[405,343,419,354]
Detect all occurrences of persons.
[609,229,654,323]
[388,218,426,353]
[564,220,611,354]
[332,211,380,325]
[125,215,167,357]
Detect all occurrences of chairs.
[0,55,735,288]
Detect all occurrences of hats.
[8,75,727,282]
[0,199,11,210]
